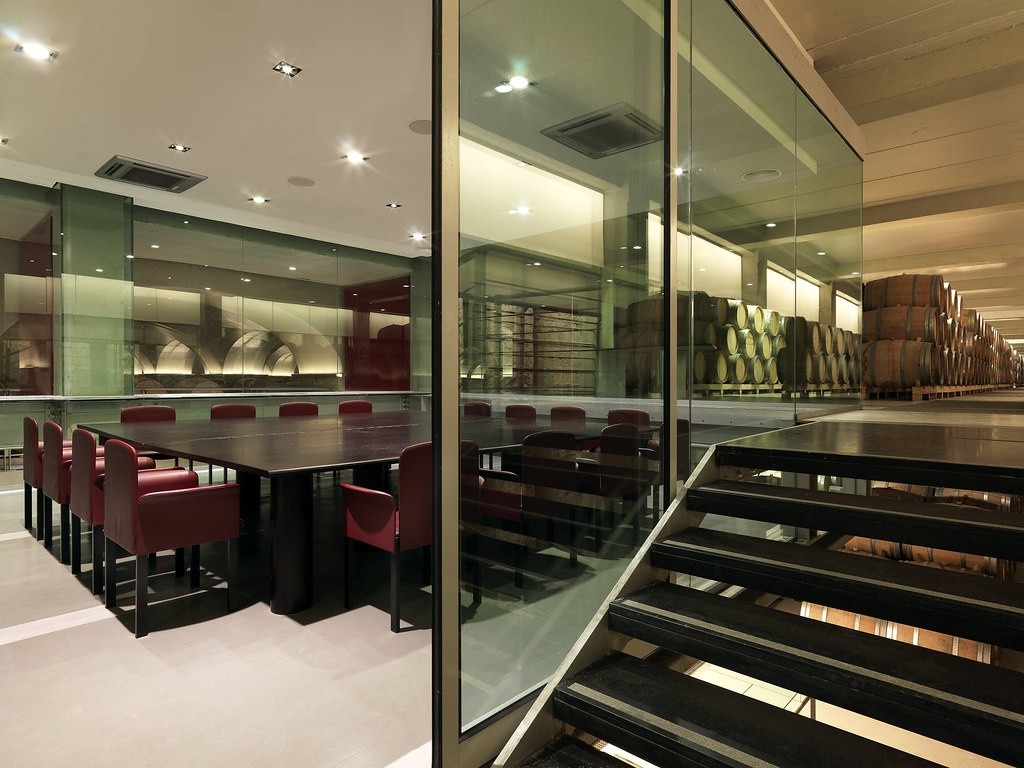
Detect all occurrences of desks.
[76,410,661,615]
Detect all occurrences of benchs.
[0,444,23,471]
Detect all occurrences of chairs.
[339,439,482,633]
[464,402,649,420]
[120,406,178,467]
[208,404,257,486]
[576,423,642,553]
[104,439,241,639]
[70,427,199,595]
[41,420,156,563]
[279,402,320,481]
[334,400,373,479]
[639,419,691,530]
[478,430,583,588]
[22,416,104,541]
